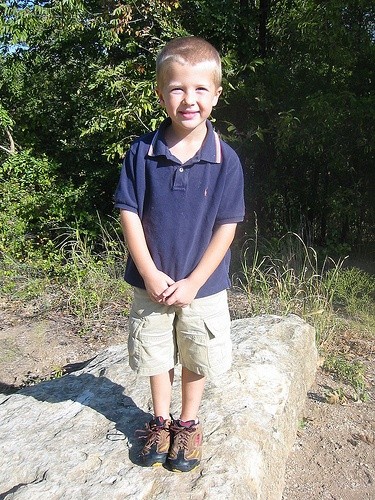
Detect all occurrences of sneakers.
[133,413,203,473]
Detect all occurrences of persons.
[115,37,245,472]
